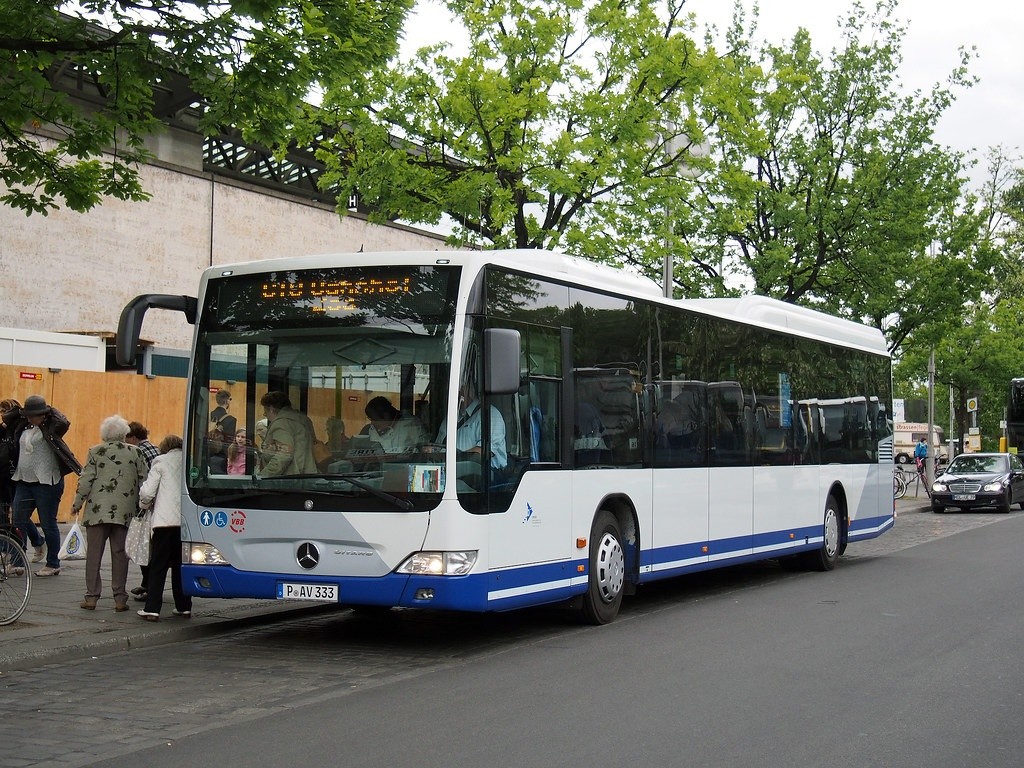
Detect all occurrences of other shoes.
[116,601,129,612]
[31,541,46,563]
[134,592,148,602]
[136,610,159,620]
[0,552,12,565]
[80,599,96,610]
[131,585,146,594]
[172,606,191,618]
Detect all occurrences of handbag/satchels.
[56,512,88,561]
[125,507,152,566]
[307,417,331,473]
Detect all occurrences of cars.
[929,453,1023,515]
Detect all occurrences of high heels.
[35,566,61,577]
[1,563,25,575]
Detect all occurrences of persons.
[125,421,162,601]
[326,416,348,445]
[421,378,507,469]
[915,438,927,475]
[2,395,84,576]
[208,390,237,475]
[961,459,976,471]
[365,395,428,452]
[227,427,247,475]
[71,415,150,612]
[256,418,268,440]
[137,434,192,622]
[0,399,48,565]
[257,392,318,477]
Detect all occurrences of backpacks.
[207,413,230,454]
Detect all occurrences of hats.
[18,395,51,415]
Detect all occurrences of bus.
[1008,377,1024,455]
[114,247,897,624]
[894,423,945,464]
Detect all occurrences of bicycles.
[895,453,945,500]
[0,527,33,626]
